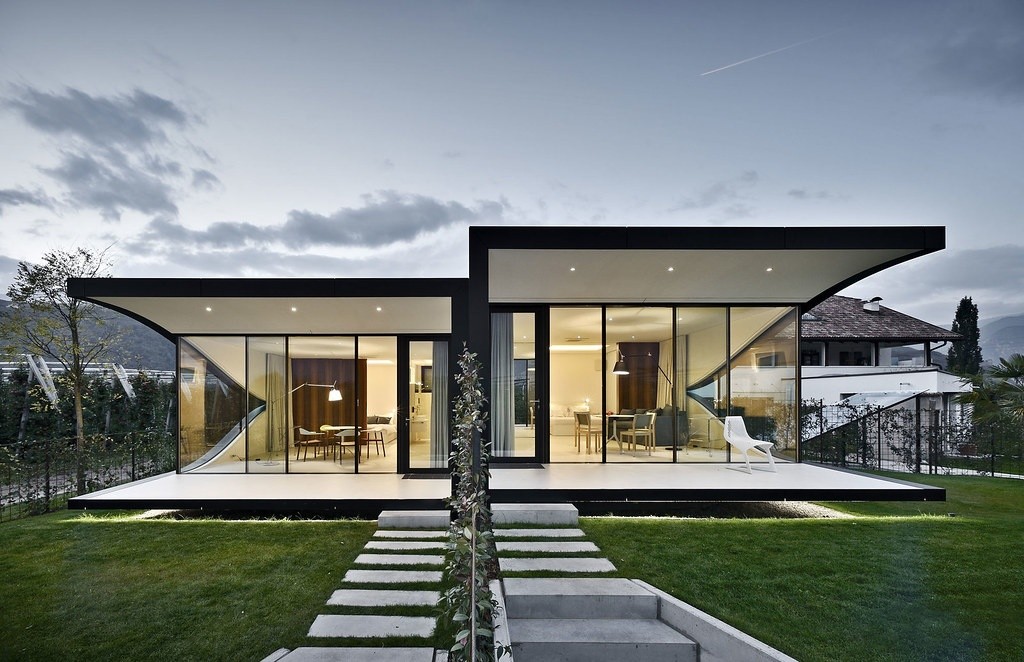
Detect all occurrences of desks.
[316,424,361,460]
[688,417,725,457]
[594,414,635,454]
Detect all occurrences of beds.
[366,424,397,444]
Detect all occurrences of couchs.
[608,408,689,446]
[550,402,618,436]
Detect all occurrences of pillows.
[367,416,392,424]
[569,405,590,417]
[550,404,569,417]
[618,404,679,415]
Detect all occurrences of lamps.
[612,350,682,451]
[268,379,343,465]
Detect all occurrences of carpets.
[490,463,545,470]
[402,473,450,479]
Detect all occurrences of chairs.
[723,416,777,474]
[529,407,536,430]
[296,425,386,462]
[573,411,602,454]
[619,411,657,456]
